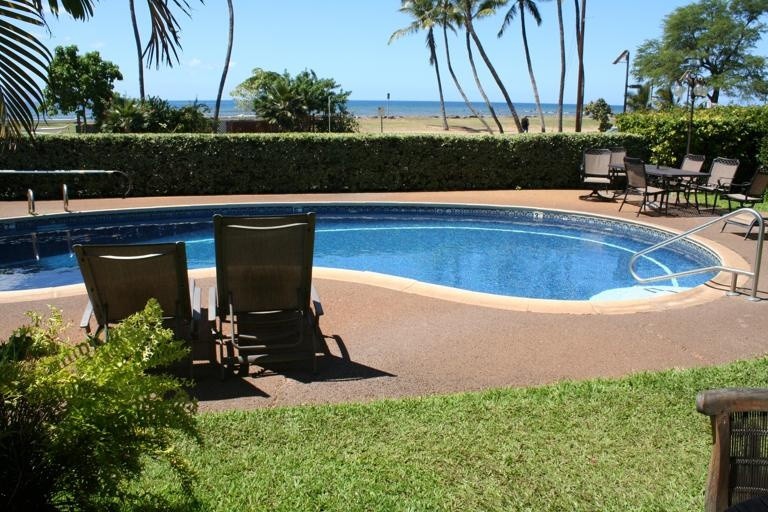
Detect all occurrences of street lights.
[327,93,334,131]
[611,48,630,114]
[387,92,391,117]
[675,69,707,155]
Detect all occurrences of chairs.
[579,147,768,241]
[71,241,201,378]
[695,387,767,512]
[208,211,324,380]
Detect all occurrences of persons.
[520,115,529,133]
